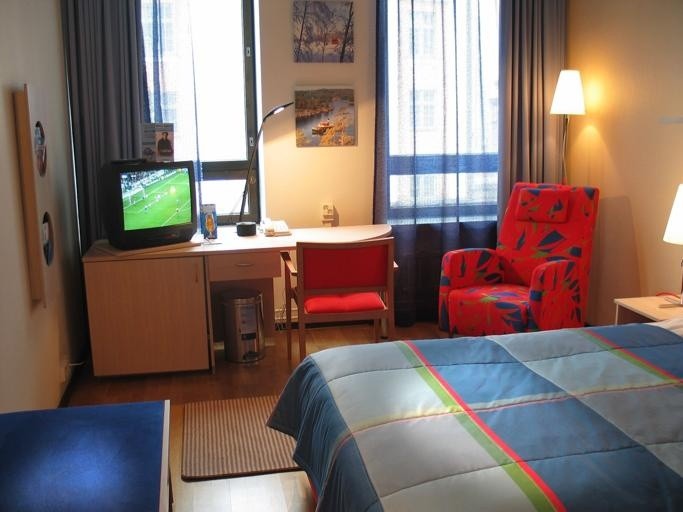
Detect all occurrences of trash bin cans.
[220,288,265,363]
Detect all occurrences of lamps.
[236,101,294,235]
[663,183,683,305]
[548,69,586,183]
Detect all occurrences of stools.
[0,400,173,512]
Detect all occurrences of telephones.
[262,217,292,236]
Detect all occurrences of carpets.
[181,390,305,479]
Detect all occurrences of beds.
[263,312,683,512]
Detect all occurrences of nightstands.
[613,294,683,324]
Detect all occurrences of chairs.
[280,234,400,364]
[436,180,600,334]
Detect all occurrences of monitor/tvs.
[108,160,197,250]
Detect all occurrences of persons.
[158,132,172,158]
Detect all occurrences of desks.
[81,223,393,376]
